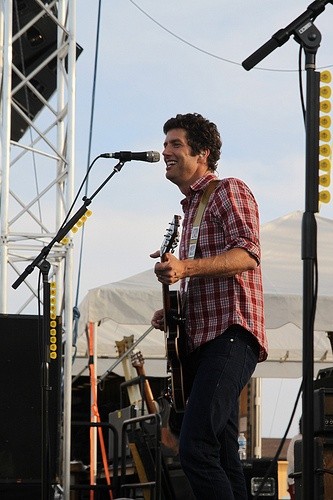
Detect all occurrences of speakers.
[0,314,63,483]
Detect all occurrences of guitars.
[113,333,160,415]
[158,215,188,412]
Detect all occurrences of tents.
[70,210,333,379]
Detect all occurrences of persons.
[151,113,269,500]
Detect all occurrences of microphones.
[100,151,160,163]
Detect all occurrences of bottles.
[238,433,246,460]
